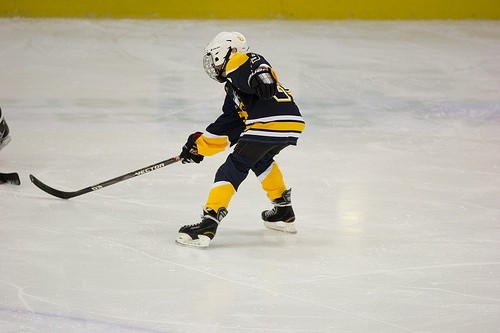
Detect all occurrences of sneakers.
[0,107,12,150]
[261,187,297,233]
[176,206,229,246]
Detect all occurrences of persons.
[0,107,21,187]
[175,31,306,248]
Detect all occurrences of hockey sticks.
[0,172,21,186]
[29,148,198,201]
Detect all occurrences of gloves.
[179,130,204,164]
[247,69,277,99]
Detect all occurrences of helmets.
[202,30,249,83]
